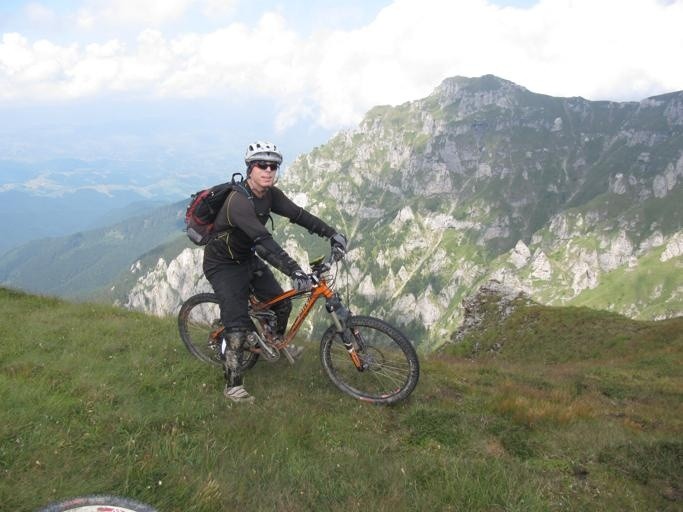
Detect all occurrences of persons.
[201,141,349,405]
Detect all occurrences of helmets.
[243,140,284,166]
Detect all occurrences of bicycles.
[176,230,419,407]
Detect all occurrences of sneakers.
[286,343,304,359]
[223,385,255,404]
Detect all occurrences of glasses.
[253,161,280,170]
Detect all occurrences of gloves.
[329,232,348,252]
[292,269,311,291]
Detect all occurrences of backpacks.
[182,172,259,247]
